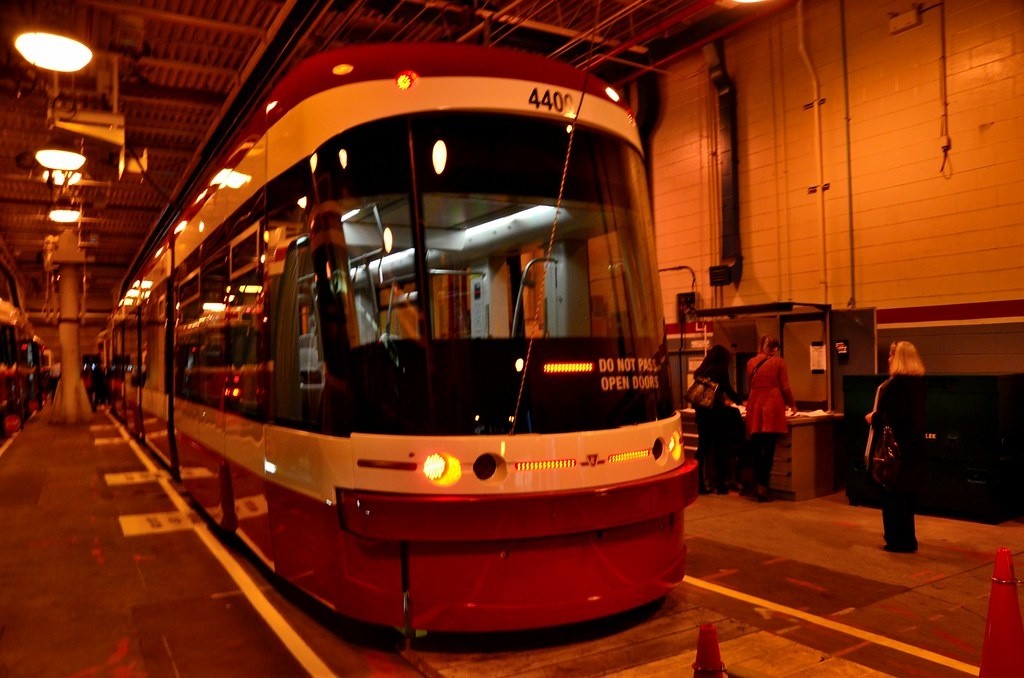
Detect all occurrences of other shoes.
[752,493,768,502]
[718,489,727,494]
[884,544,918,553]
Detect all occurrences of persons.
[691,345,747,494]
[864,340,926,553]
[743,335,797,500]
[83,361,107,412]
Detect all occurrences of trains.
[0,239,53,434]
[93,42,703,633]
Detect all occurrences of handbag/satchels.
[870,424,901,491]
[682,375,719,408]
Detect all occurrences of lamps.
[34,143,87,171]
